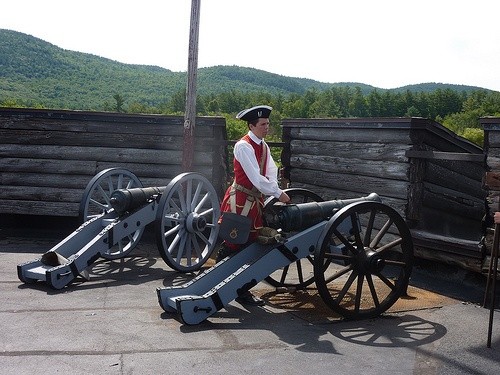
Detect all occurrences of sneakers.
[235,290,266,306]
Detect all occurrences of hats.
[236,105,273,121]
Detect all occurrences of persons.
[215,105,290,305]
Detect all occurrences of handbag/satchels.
[218,212,253,244]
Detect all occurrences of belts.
[232,182,262,197]
